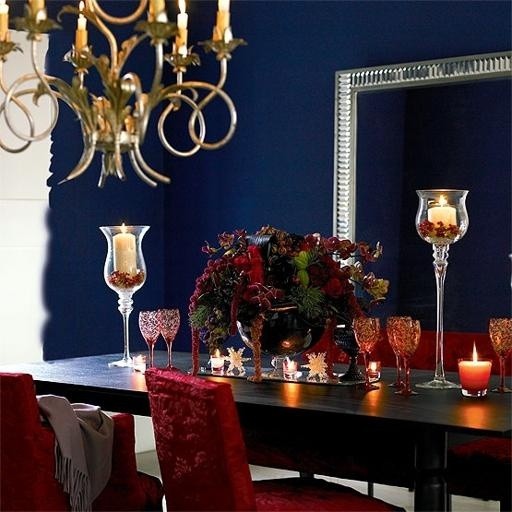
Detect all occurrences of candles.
[428,196,457,228]
[113,223,138,276]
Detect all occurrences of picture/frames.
[332,51,512,295]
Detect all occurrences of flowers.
[188,225,390,382]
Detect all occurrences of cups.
[133,355,147,372]
[282,360,297,375]
[368,361,381,371]
[210,354,225,376]
[457,360,492,398]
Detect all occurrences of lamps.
[0,0,249,188]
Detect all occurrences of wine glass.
[157,308,181,371]
[487,316,512,393]
[414,186,470,389]
[138,310,162,368]
[98,224,152,371]
[353,315,382,391]
[385,315,413,388]
[391,319,422,396]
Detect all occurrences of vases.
[236,305,325,380]
